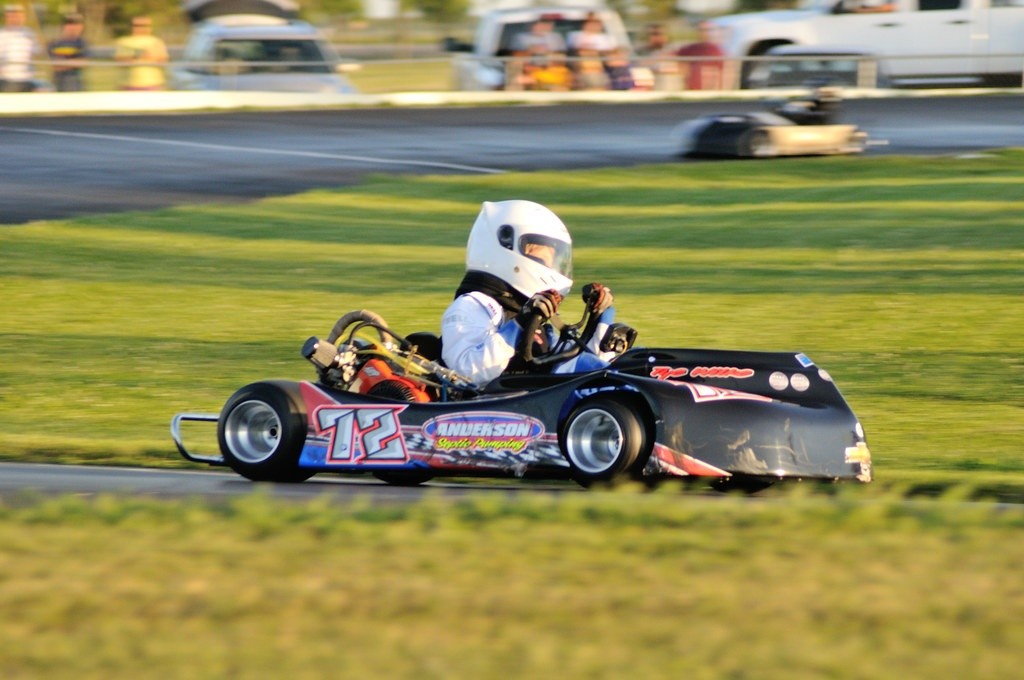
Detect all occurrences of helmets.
[465,200,574,304]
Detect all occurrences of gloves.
[515,289,562,331]
[581,282,614,317]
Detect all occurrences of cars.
[166,2,356,93]
[455,2,662,92]
[675,1,1022,89]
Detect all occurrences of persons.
[0,3,169,90]
[502,17,723,94]
[440,200,614,393]
